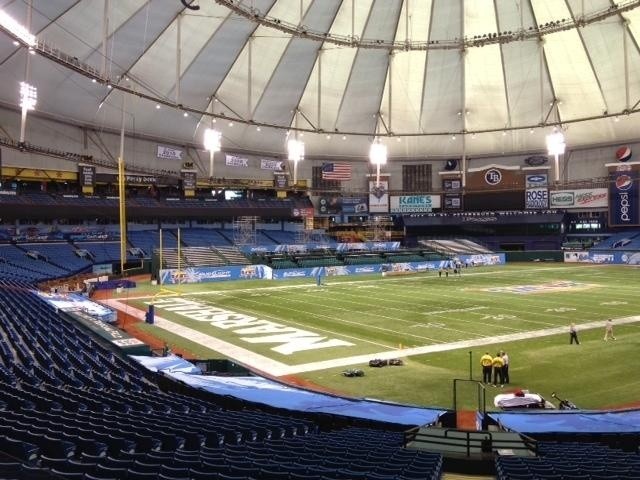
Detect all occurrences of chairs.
[495,433,638,478]
[0,192,121,279]
[3,388,445,480]
[1,282,159,394]
[590,231,639,251]
[333,234,494,265]
[125,194,344,277]
[402,210,570,236]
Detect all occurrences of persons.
[501,350,511,384]
[437,258,476,277]
[479,351,493,385]
[603,318,617,342]
[569,322,582,346]
[491,353,506,388]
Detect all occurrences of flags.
[321,161,353,181]
